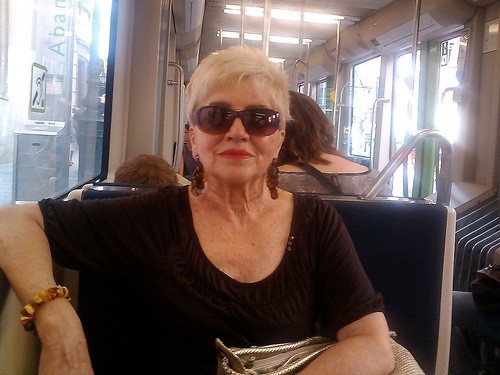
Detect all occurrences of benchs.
[48,183,458,375]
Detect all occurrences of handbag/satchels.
[215,332,425,375]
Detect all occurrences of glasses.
[195,106,279,137]
